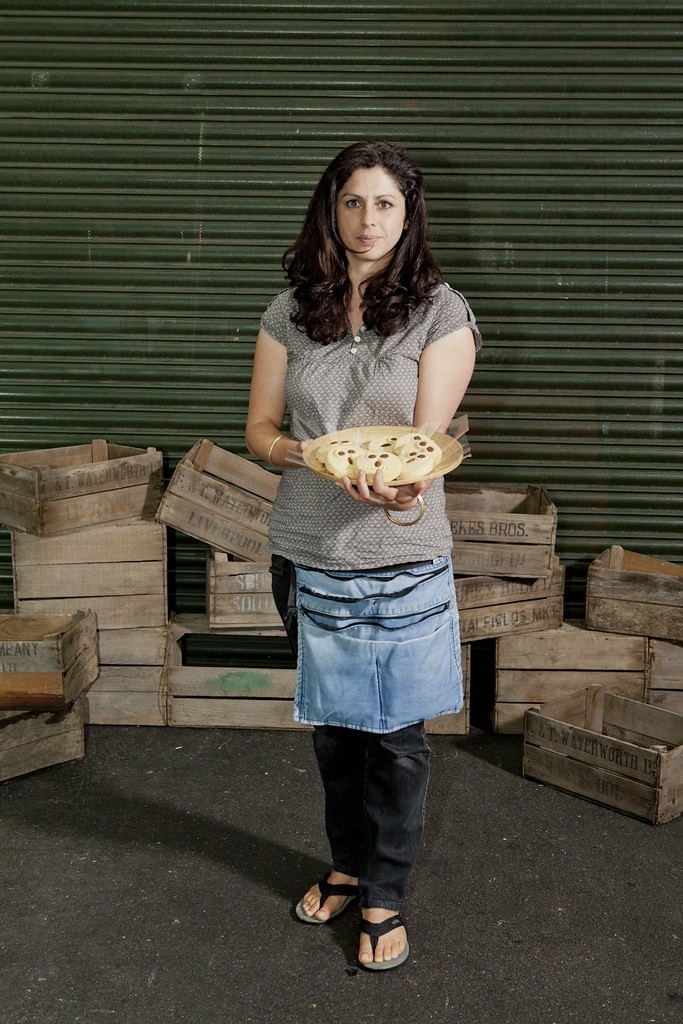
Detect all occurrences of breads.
[315,433,443,481]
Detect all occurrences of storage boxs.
[1,439,682,827]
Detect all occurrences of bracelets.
[381,494,426,527]
[242,141,484,969]
[267,434,287,469]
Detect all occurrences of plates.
[303,425,463,487]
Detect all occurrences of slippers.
[359,912,411,969]
[294,874,362,925]
